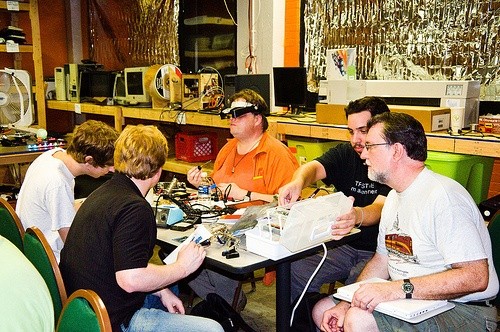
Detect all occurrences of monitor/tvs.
[274,67,307,116]
[112,74,138,106]
[124,67,152,108]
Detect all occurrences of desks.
[76,186,362,332]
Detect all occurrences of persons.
[278,96,407,316]
[166,88,303,311]
[14,118,121,263]
[312,113,500,332]
[57,124,227,332]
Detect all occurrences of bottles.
[198,173,210,210]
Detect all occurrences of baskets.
[175,132,218,163]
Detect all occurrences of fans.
[0,67,48,140]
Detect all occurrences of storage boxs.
[424,150,495,205]
[287,138,349,188]
[175,130,218,163]
[316,103,451,133]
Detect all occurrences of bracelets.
[354,207,365,229]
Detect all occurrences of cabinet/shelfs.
[0,0,47,154]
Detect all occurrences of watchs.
[402,278,415,299]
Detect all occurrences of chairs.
[0,198,113,332]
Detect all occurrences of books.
[333,276,456,324]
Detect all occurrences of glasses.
[365,142,390,151]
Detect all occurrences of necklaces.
[231,138,259,174]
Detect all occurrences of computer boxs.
[223,73,270,116]
